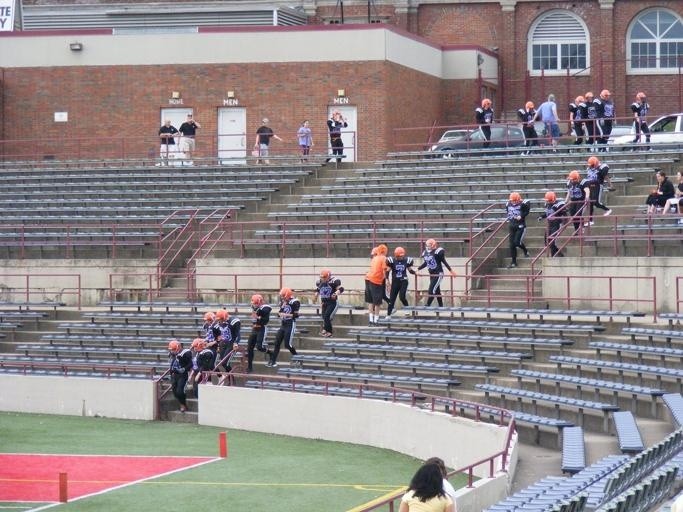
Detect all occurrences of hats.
[371,244,387,256]
[262,118,269,122]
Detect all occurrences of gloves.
[233,343,239,352]
[194,371,202,383]
[599,117,605,126]
[331,292,336,300]
[176,367,186,374]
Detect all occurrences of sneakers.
[520,143,653,157]
[180,406,188,412]
[368,321,379,327]
[380,316,392,322]
[506,263,517,268]
[603,209,612,217]
[584,221,594,227]
[265,360,278,368]
[391,307,397,314]
[318,330,332,338]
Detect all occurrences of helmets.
[585,92,594,100]
[544,191,556,202]
[567,171,580,180]
[575,95,585,107]
[320,268,331,280]
[482,98,492,109]
[203,312,215,321]
[425,238,437,250]
[509,192,521,201]
[251,294,263,307]
[588,156,599,167]
[191,338,206,352]
[333,110,340,115]
[526,101,535,109]
[167,340,182,354]
[279,287,292,301]
[394,247,405,257]
[636,92,646,103]
[216,309,229,321]
[600,89,611,101]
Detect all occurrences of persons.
[398,463,454,512]
[167,341,192,412]
[423,456,457,511]
[245,293,273,373]
[591,156,613,224]
[518,89,654,151]
[645,170,674,214]
[312,270,343,338]
[474,98,494,147]
[370,246,395,314]
[157,117,178,167]
[200,311,217,361]
[363,244,387,325]
[505,191,529,268]
[386,246,417,316]
[536,190,567,257]
[564,169,591,235]
[295,119,314,162]
[269,287,300,363]
[188,339,215,399]
[660,166,682,215]
[254,117,282,164]
[325,109,347,163]
[416,238,455,307]
[213,309,240,375]
[177,112,200,166]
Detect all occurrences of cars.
[426,111,683,161]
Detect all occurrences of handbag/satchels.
[251,145,261,157]
